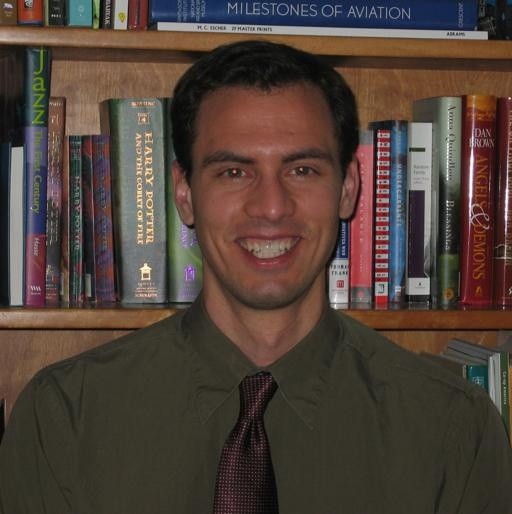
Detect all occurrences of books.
[324,95,511,311]
[1,0,512,40]
[421,337,512,433]
[2,44,206,307]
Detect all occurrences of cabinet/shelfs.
[0,25,512,438]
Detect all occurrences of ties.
[212,370,280,514]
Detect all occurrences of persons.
[2,39,512,511]
